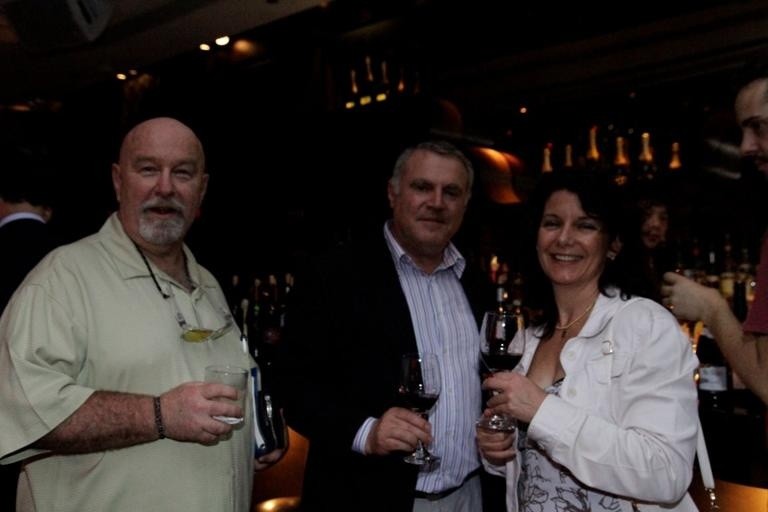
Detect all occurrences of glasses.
[163,282,235,344]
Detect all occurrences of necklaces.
[553,296,597,331]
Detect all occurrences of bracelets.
[153,395,165,440]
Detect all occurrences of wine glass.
[475,311,522,432]
[398,350,445,465]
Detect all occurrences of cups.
[201,363,249,427]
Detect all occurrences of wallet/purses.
[252,389,290,476]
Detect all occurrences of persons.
[658,54,768,406]
[626,184,672,303]
[262,136,499,512]
[1,147,55,317]
[476,166,701,512]
[0,115,262,512]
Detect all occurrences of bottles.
[675,234,761,426]
[223,272,298,363]
[538,124,683,192]
[485,258,539,330]
[345,54,414,113]
